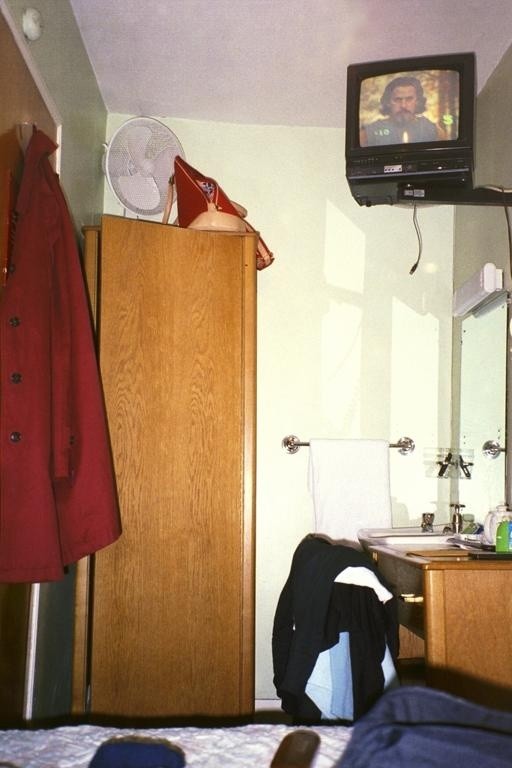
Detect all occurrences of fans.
[100,116,187,219]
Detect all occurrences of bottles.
[496,518,512,555]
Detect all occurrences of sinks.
[357,528,450,551]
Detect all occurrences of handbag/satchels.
[173,155,249,233]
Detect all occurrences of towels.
[306,437,393,541]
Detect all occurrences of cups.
[483,505,512,546]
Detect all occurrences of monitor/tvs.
[345,51,477,206]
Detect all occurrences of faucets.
[421,514,434,532]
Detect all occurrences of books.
[406,548,472,561]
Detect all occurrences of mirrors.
[455,290,510,532]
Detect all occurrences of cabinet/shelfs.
[70,212,261,722]
[364,549,512,713]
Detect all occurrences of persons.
[358,76,446,151]
